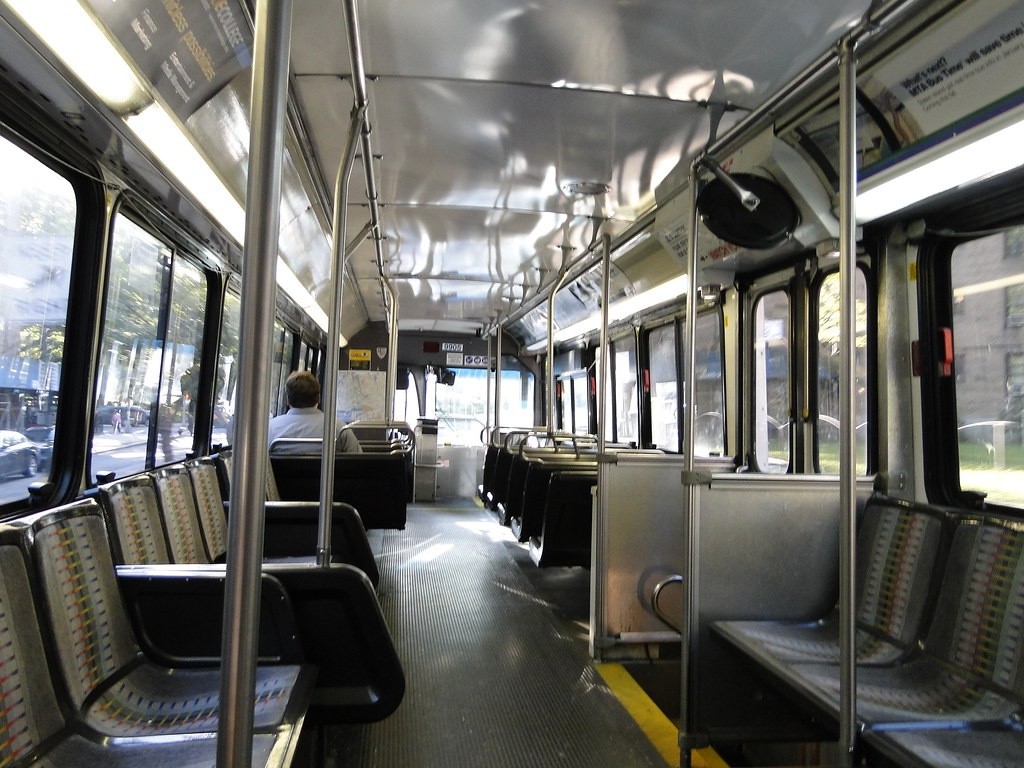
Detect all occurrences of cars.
[0,428,42,483]
[22,424,56,467]
[97,405,150,427]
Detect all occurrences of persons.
[270,371,363,451]
[176,364,231,425]
[24,400,37,429]
[113,409,122,434]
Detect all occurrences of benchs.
[0,453,406,768]
[477,425,1023,768]
